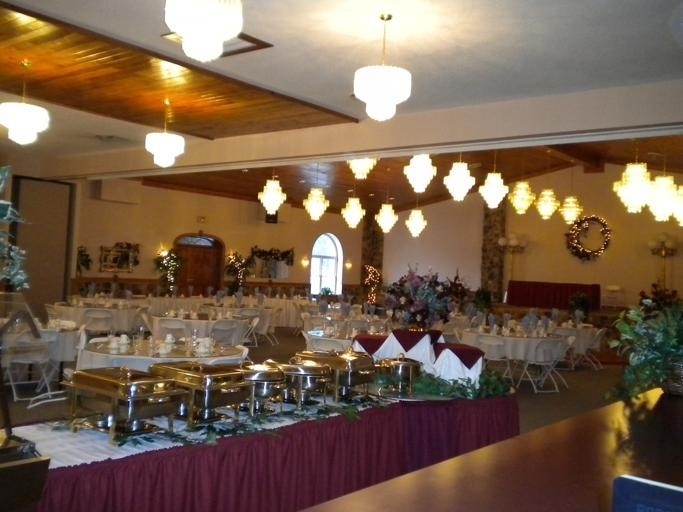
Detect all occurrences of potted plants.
[604,296,683,403]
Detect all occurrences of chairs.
[256,307,284,347]
[549,327,577,335]
[571,326,607,372]
[78,308,114,338]
[126,306,150,336]
[241,317,258,349]
[454,327,462,343]
[45,305,62,320]
[158,318,191,338]
[4,328,57,402]
[474,334,515,385]
[37,325,87,393]
[142,313,152,336]
[209,319,239,346]
[516,337,564,394]
[539,336,576,390]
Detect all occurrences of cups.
[13,318,21,332]
[132,335,142,349]
[191,328,199,347]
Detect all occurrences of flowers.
[383,261,471,330]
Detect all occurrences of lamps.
[404,154,437,193]
[259,168,285,215]
[0,58,50,144]
[499,234,529,280]
[374,193,398,233]
[673,185,683,227]
[346,160,377,179]
[164,1,243,62]
[145,97,185,168]
[646,148,678,222]
[354,13,410,121]
[303,161,329,221]
[559,163,583,225]
[479,150,509,210]
[405,193,428,238]
[341,180,366,228]
[508,181,536,215]
[648,232,676,290]
[443,152,475,202]
[612,142,656,213]
[534,149,561,220]
